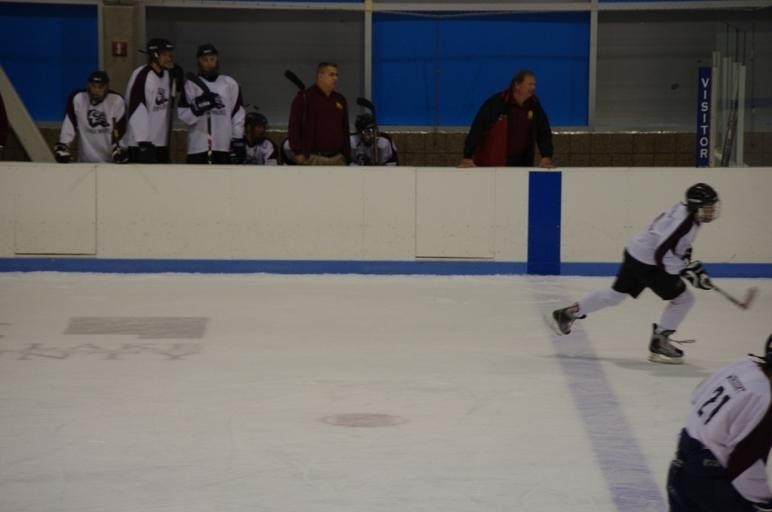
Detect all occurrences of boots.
[554,302,585,335]
[649,322,684,358]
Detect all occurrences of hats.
[86,71,110,86]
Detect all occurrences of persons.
[666,333,772,512]
[553,183,722,358]
[458,71,558,169]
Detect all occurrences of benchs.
[1,123,771,172]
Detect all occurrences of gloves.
[680,260,713,290]
[189,91,225,116]
[229,137,248,163]
[111,144,129,163]
[53,141,73,163]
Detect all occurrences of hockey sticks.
[184,71,212,163]
[357,96,378,165]
[283,69,307,163]
[713,283,758,310]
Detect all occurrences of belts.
[310,149,341,158]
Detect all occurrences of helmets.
[146,38,176,53]
[244,112,268,128]
[355,114,375,134]
[196,43,218,58]
[686,183,720,212]
